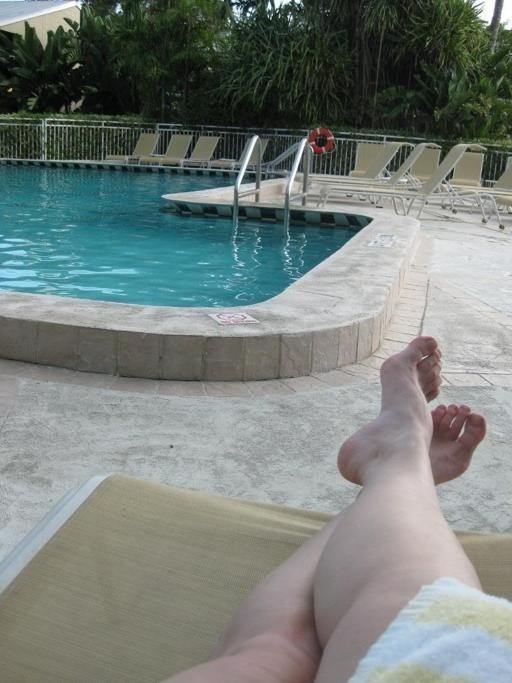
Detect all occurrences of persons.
[147,334,511,682]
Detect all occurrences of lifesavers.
[308,126,334,154]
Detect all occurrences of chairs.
[292,141,511,227]
[105,133,269,171]
[0,472,512,682]
